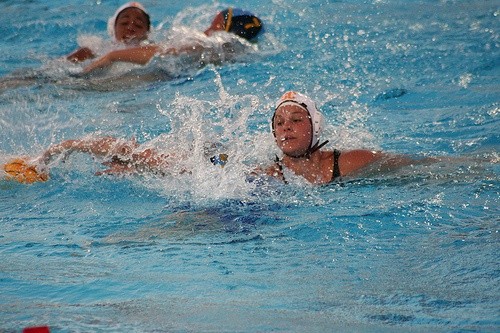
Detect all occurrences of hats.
[0,157,50,184]
[268,91,324,148]
[221,7,262,39]
[107,2,150,42]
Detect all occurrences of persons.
[1,2,266,240]
[245,90,492,192]
